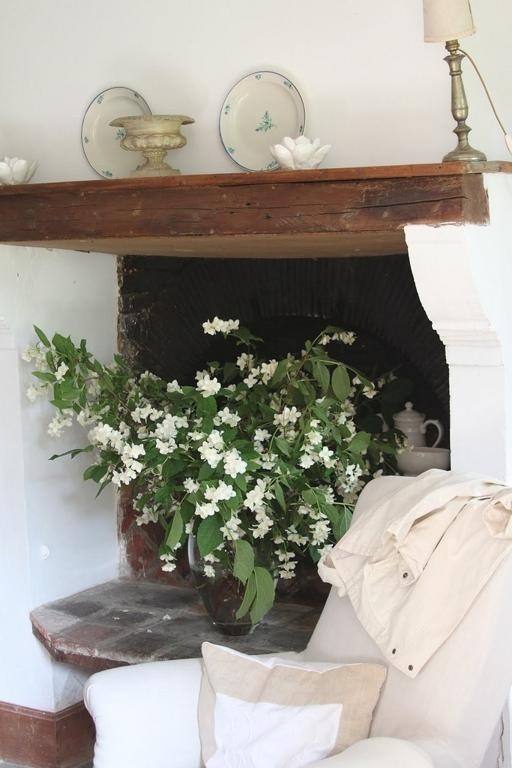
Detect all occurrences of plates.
[218,67,306,171]
[79,84,154,177]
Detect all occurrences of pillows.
[197,641,387,768]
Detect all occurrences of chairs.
[82,474,512,768]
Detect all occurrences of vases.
[187,522,282,636]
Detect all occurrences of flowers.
[21,316,408,625]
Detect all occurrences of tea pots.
[374,400,443,448]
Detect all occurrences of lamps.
[423,0,487,162]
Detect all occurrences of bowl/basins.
[394,447,450,476]
